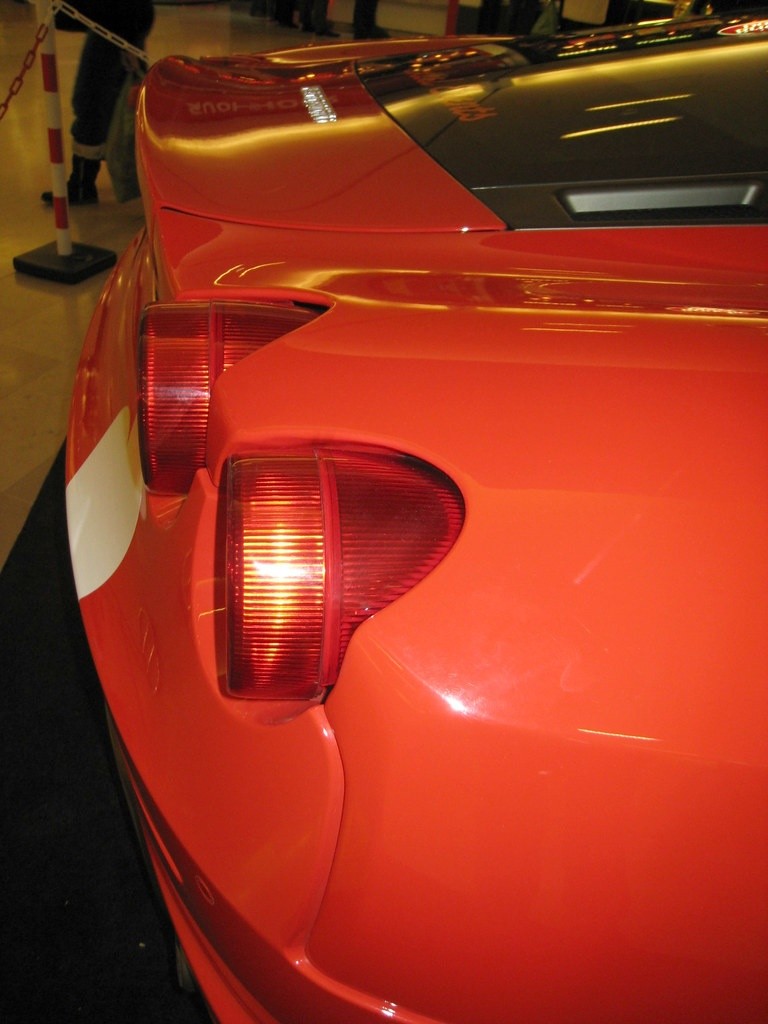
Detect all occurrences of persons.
[691,0,768,16]
[478,0,561,36]
[352,0,388,39]
[41,0,155,203]
[603,0,673,27]
[250,0,340,37]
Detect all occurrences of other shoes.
[41,180,98,205]
[250,7,392,39]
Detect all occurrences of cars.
[64,3,768,1024]
